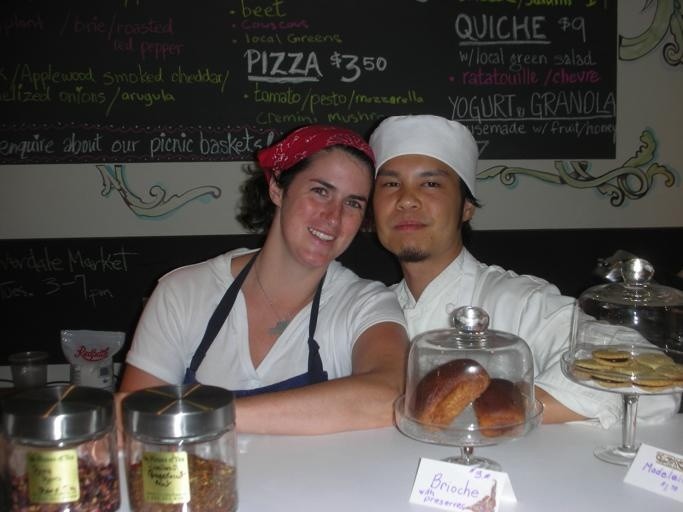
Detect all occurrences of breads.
[473,379,526,437]
[408,357,491,432]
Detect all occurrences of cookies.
[574,351,683,393]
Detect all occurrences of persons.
[119,125,411,437]
[367,114,683,428]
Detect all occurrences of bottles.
[0,384,123,512]
[120,383,239,512]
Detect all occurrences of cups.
[8,350,48,387]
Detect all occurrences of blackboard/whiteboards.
[0,225,683,366]
[0,0,618,165]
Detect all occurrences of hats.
[368,113,477,197]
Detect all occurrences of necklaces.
[253,259,317,333]
